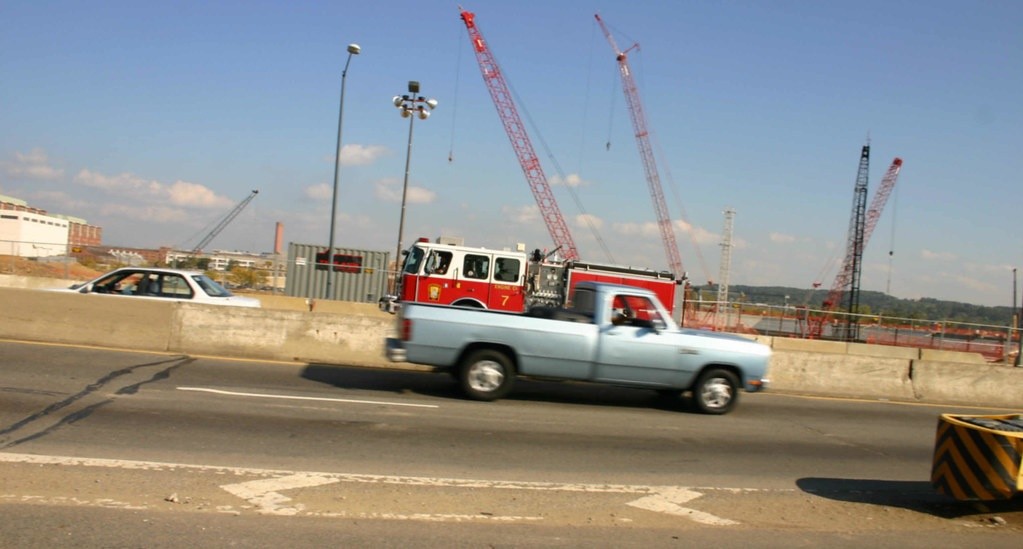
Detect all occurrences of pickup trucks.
[382,281,775,419]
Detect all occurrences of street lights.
[326,42,360,300]
[383,77,440,299]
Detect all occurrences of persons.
[435,250,452,274]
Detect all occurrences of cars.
[48,267,261,309]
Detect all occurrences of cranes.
[456,6,757,334]
[790,137,904,339]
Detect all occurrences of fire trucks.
[376,236,687,346]
